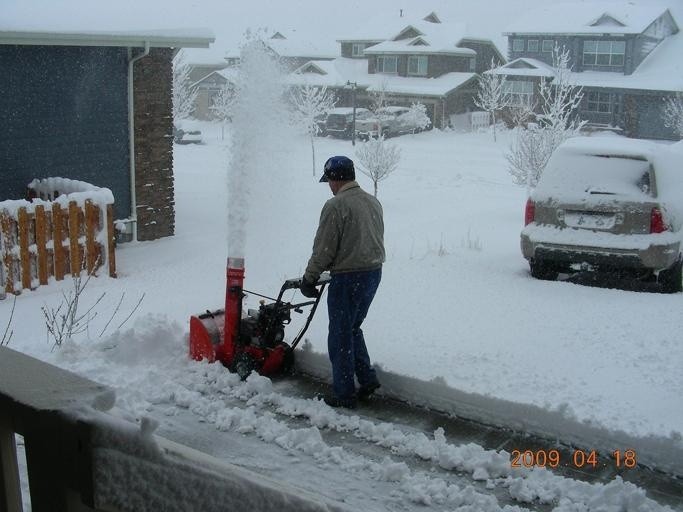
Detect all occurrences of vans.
[322,107,373,140]
[518,133,683,293]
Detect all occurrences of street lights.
[343,80,361,147]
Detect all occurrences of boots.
[324,390,357,410]
[356,367,380,401]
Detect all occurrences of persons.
[298,155,386,410]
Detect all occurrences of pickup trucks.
[352,106,421,138]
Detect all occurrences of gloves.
[300,275,321,299]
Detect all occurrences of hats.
[318,155,356,183]
